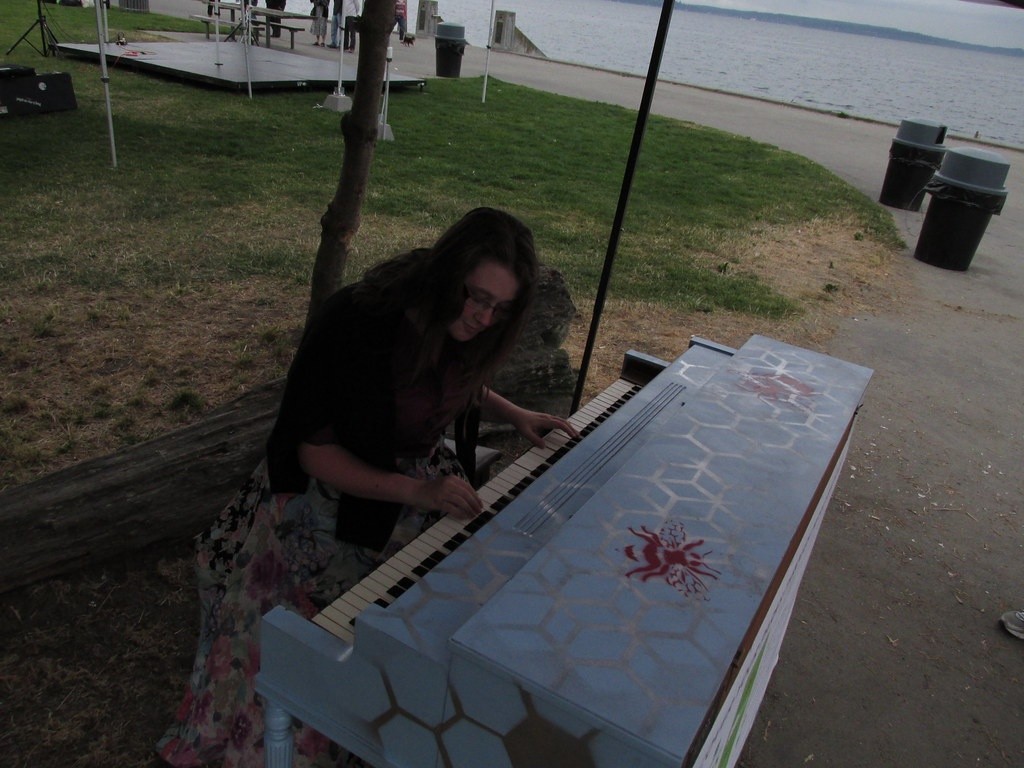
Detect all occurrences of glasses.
[463,294,513,321]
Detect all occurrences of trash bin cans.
[913,147,1011,272]
[435,22,465,78]
[879,119,949,213]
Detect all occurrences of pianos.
[249,333,874,768]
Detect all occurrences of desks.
[203,1,320,47]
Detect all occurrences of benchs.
[443,438,501,489]
[239,17,306,50]
[191,14,265,41]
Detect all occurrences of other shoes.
[349,48,355,53]
[327,43,339,49]
[312,42,320,46]
[270,35,281,38]
[320,43,326,47]
[344,47,351,52]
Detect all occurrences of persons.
[327,0,343,47]
[243,0,258,19]
[207,0,220,18]
[390,0,407,43]
[155,207,579,768]
[310,0,330,46]
[345,0,363,53]
[265,0,286,37]
